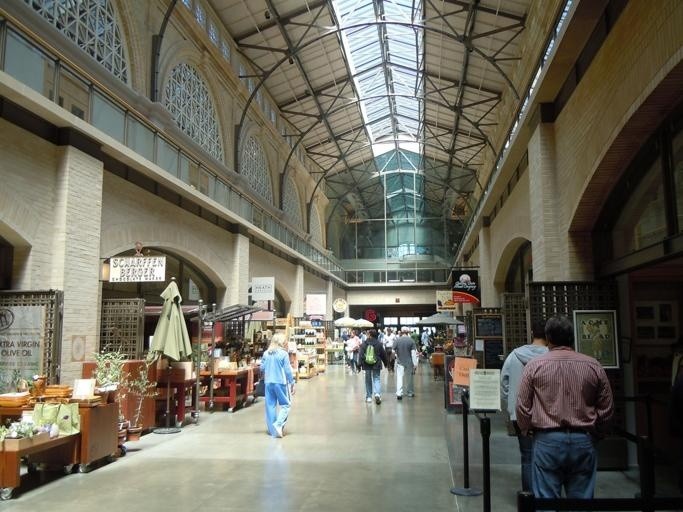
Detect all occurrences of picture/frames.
[573,310,620,369]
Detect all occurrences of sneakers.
[407,392,416,398]
[397,394,402,400]
[272,423,284,438]
[375,393,382,405]
[364,397,373,404]
[345,364,362,376]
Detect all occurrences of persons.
[581,319,610,359]
[515,315,614,512]
[259,332,295,437]
[341,327,438,404]
[501,318,547,492]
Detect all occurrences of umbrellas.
[354,318,374,328]
[245,303,268,339]
[415,312,464,330]
[151,278,193,428]
[334,316,356,327]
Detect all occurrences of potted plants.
[96,342,159,443]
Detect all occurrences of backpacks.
[364,340,380,364]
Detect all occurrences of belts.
[534,425,594,435]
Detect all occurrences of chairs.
[429,353,445,382]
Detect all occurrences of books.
[469,369,502,411]
[452,357,477,386]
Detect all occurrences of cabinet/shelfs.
[290,315,326,378]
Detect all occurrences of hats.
[401,326,409,333]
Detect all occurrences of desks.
[326,347,346,366]
[156,379,196,422]
[0,388,119,501]
[198,365,259,408]
